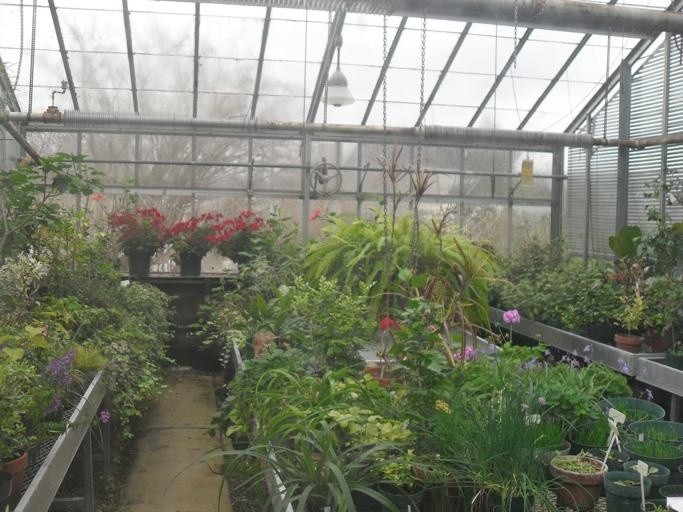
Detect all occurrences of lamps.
[322,35,356,106]
